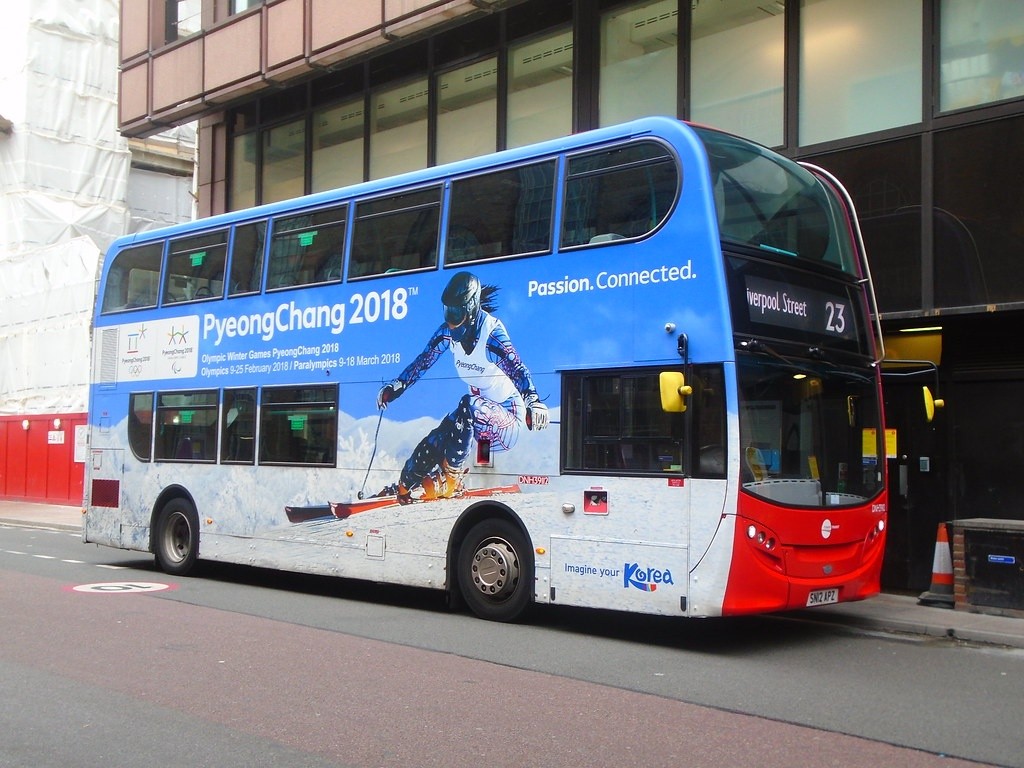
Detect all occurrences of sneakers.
[410,457,469,500]
[378,477,410,497]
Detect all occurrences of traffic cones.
[917,522,954,609]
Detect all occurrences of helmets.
[441,271,481,341]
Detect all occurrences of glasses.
[443,304,467,325]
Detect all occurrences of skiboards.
[284,481,522,526]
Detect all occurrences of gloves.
[523,390,550,431]
[376,378,407,411]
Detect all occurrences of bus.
[82,116,944,625]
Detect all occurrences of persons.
[377,272,549,506]
